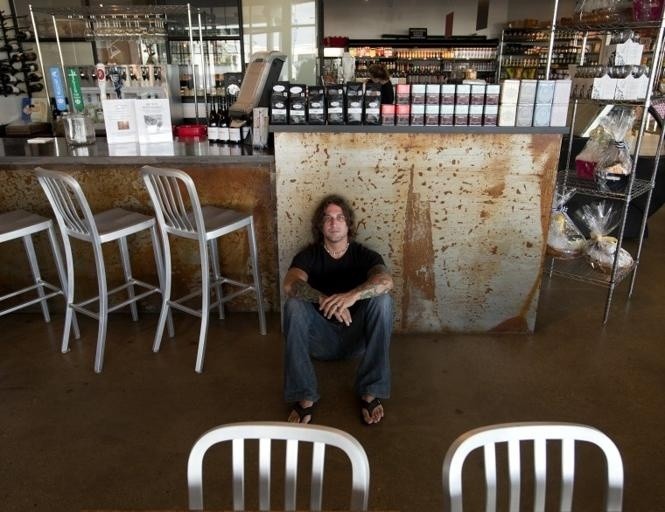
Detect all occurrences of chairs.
[440,421,625,511]
[185,420,372,511]
[139,164,269,376]
[33,166,175,375]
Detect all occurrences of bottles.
[209,96,218,143]
[353,60,447,84]
[397,49,447,60]
[502,32,597,68]
[448,46,497,73]
[381,83,500,127]
[573,82,593,99]
[219,96,229,146]
[537,69,569,79]
[0,30,44,96]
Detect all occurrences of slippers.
[293,402,314,424]
[360,397,384,427]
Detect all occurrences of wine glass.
[82,17,168,44]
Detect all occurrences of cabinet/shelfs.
[496,25,603,81]
[343,37,496,84]
[546,0,665,329]
[167,36,247,105]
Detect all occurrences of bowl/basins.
[176,125,207,137]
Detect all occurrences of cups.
[61,113,97,147]
[646,113,659,132]
[506,67,536,80]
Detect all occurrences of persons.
[282,197,393,425]
[367,64,394,105]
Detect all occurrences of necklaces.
[324,242,349,255]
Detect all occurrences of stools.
[0,208,84,340]
